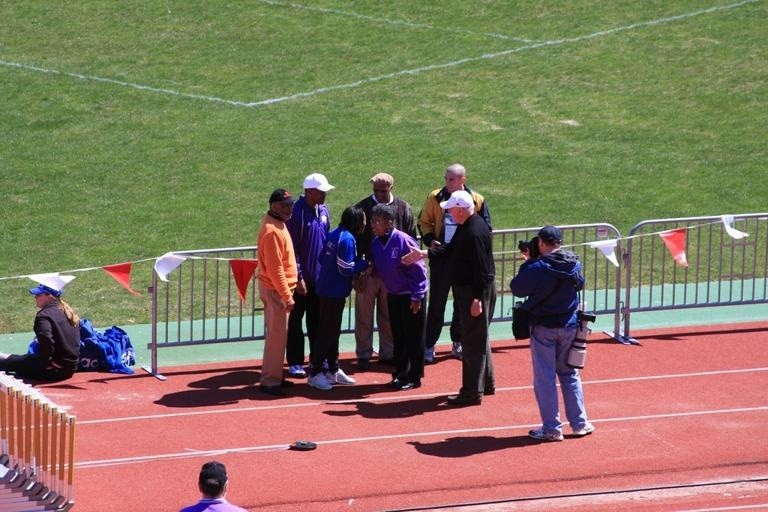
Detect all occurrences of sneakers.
[572,422,595,435]
[528,428,563,441]
[259,342,496,405]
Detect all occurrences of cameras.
[519,237,542,258]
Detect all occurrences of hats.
[538,225,563,243]
[303,173,336,192]
[269,188,292,203]
[440,190,473,208]
[198,462,227,489]
[368,171,393,185]
[30,284,61,297]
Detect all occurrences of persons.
[178,461,249,512]
[307,206,374,391]
[416,163,494,365]
[0,276,80,384]
[253,187,300,397]
[287,172,332,376]
[508,224,596,442]
[361,204,430,391]
[350,170,418,370]
[399,189,498,407]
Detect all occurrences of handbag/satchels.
[28,320,135,371]
[512,301,531,340]
